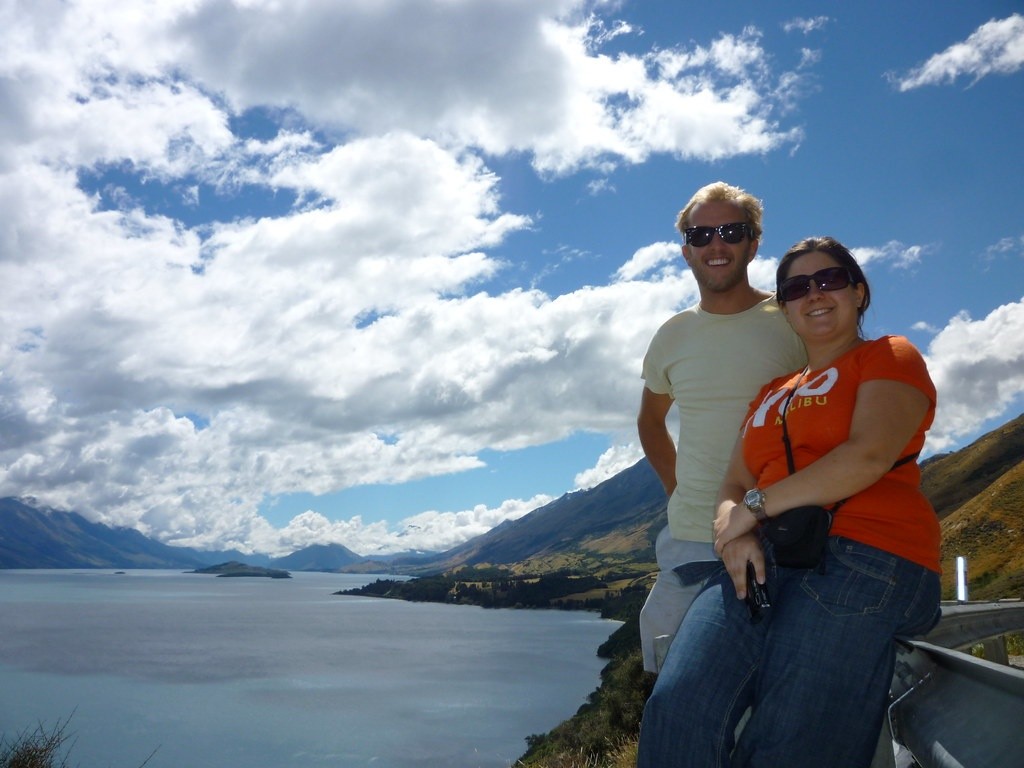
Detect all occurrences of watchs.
[743,487,767,522]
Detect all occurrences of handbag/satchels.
[768,505,833,575]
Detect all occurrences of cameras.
[745,562,771,628]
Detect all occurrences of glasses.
[684,222,751,247]
[778,266,857,301]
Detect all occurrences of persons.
[636,181,809,766]
[635,236,942,768]
